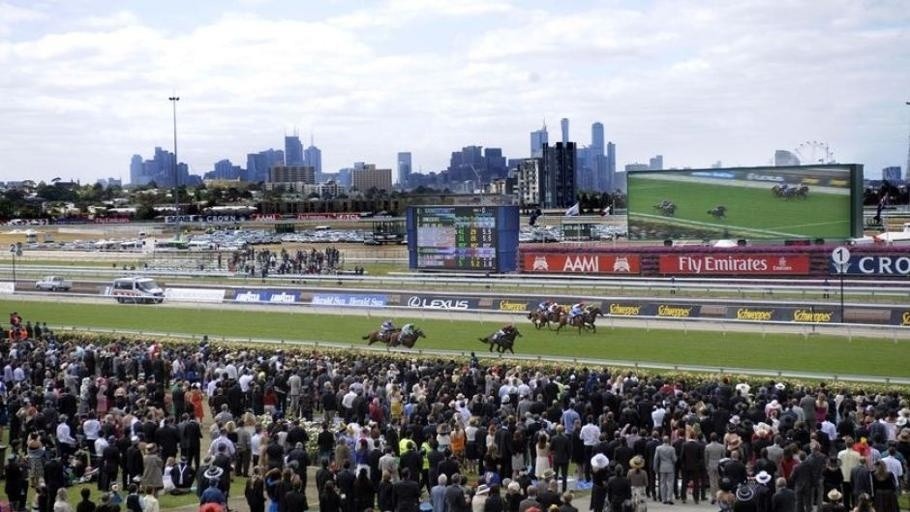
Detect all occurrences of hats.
[204,466,224,480]
[436,423,452,434]
[456,392,466,399]
[107,435,159,454]
[727,382,910,503]
[475,453,645,512]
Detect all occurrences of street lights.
[168,96,182,239]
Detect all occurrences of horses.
[362,328,401,346]
[771,185,809,201]
[653,200,670,208]
[663,204,677,215]
[706,206,728,220]
[478,328,522,354]
[386,328,426,347]
[530,307,604,336]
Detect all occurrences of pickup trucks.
[35,275,72,291]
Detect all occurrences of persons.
[822,278,831,298]
[546,302,558,314]
[0,313,909,510]
[669,276,677,294]
[568,301,587,319]
[782,181,788,193]
[216,244,365,287]
[537,298,550,312]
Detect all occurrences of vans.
[112,277,165,304]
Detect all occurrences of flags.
[872,194,889,223]
[565,202,579,216]
[599,203,613,217]
[528,209,542,225]
